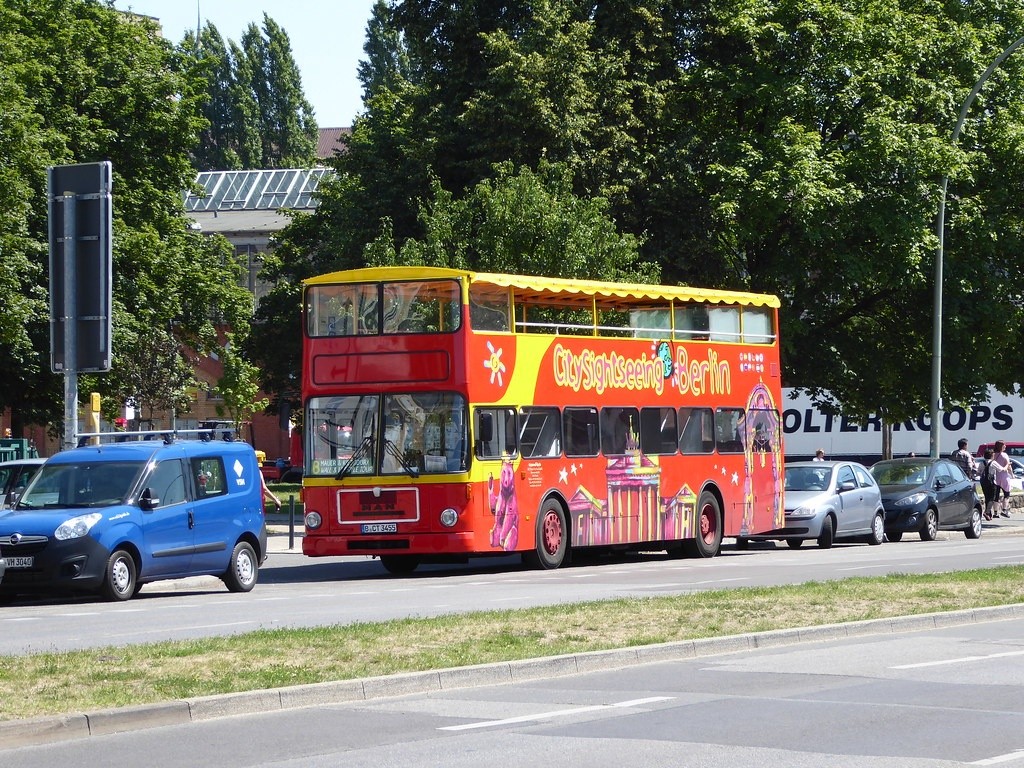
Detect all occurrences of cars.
[1,458,68,516]
[867,459,983,543]
[734,461,887,551]
[970,456,1024,497]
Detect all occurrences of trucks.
[260,417,358,494]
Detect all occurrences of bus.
[976,441,1024,475]
[277,267,788,557]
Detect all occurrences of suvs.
[0,428,270,609]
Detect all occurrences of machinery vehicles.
[0,426,41,491]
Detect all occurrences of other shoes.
[985,509,1010,521]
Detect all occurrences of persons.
[948,439,973,483]
[975,450,1013,520]
[993,440,1015,519]
[257,469,282,561]
[811,449,920,486]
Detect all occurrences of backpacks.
[981,459,995,487]
